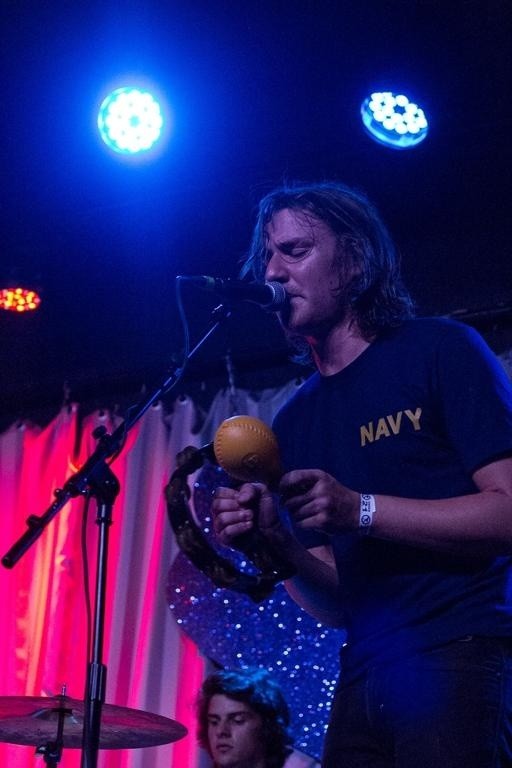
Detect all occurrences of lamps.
[95,86,168,158]
[358,59,435,151]
[1,261,45,314]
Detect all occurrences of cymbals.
[1,696,188,750]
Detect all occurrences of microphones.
[200,276,286,312]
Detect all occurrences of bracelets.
[350,487,378,537]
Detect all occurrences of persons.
[201,184,509,767]
[195,666,303,767]
